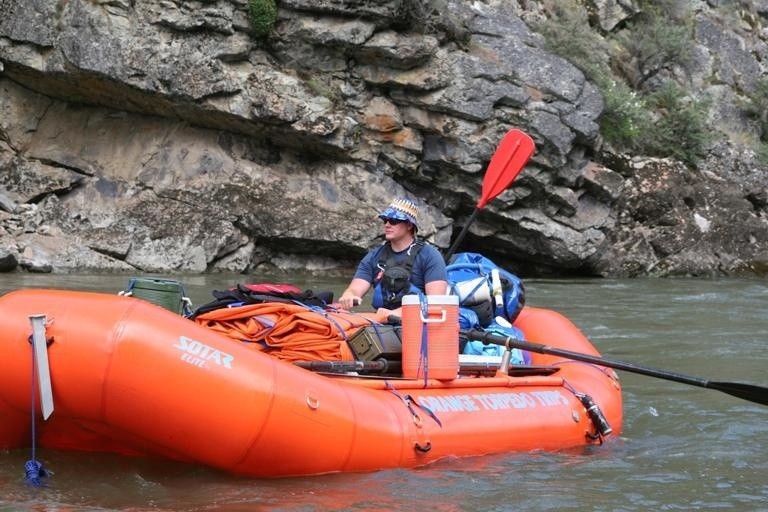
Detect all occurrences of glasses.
[382,218,409,226]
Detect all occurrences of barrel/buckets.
[130,279,182,314]
[401,294,460,380]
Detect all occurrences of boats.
[0,288,624,479]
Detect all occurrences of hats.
[379,198,420,234]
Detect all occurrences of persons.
[338,197,448,324]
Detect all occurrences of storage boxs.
[346,324,402,362]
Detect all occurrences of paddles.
[444,129,535,263]
[294,360,560,377]
[387,313,768,406]
